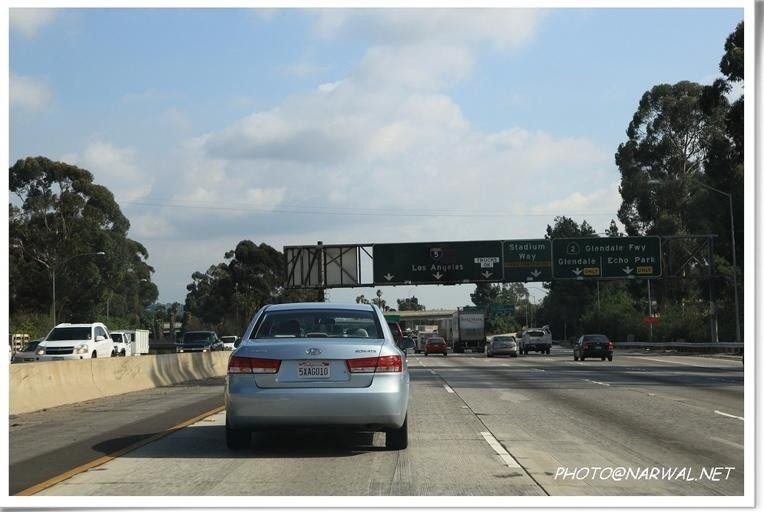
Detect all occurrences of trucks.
[449,306,487,353]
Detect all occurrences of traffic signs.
[373,236,664,286]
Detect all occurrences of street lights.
[9,244,107,331]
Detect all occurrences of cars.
[10,322,151,362]
[219,335,238,350]
[572,333,615,362]
[486,335,519,359]
[519,326,554,355]
[224,300,416,452]
[403,330,448,356]
[174,331,224,353]
